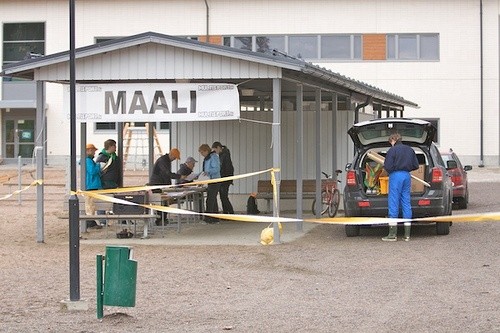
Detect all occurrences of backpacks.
[247,195,260,214]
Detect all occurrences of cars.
[440,148,472,209]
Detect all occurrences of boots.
[382,222,397,241]
[402,222,411,242]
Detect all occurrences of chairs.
[416,153,426,164]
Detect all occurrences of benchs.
[254,178,337,213]
[57,194,161,238]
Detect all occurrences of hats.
[171,147,181,160]
[186,156,198,162]
[86,143,97,150]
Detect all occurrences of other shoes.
[155,220,168,225]
[204,217,219,222]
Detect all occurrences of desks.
[106,188,205,236]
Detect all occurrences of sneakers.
[87,220,103,229]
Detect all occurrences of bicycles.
[312,170,341,218]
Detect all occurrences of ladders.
[119,123,163,177]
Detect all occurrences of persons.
[149,141,234,226]
[85,139,120,229]
[382,133,420,242]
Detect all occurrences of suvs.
[345,118,454,237]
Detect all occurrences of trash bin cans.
[96,245,137,319]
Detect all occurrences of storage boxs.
[113,191,148,215]
